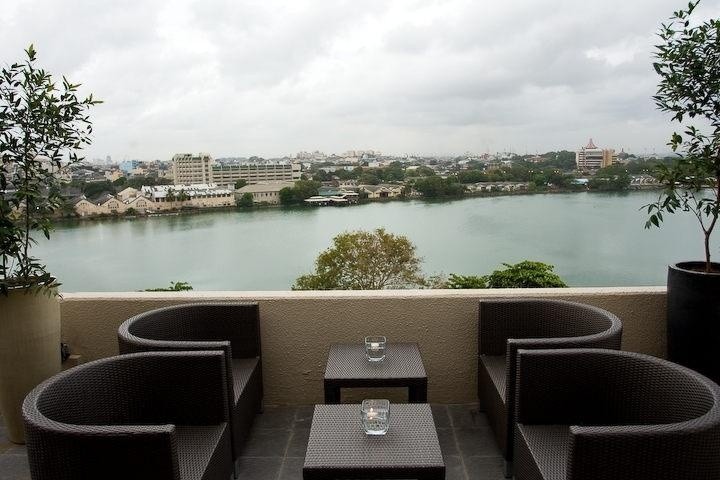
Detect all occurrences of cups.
[361,399,390,436]
[365,335,386,363]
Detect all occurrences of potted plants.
[0,43,106,445]
[636,0,720,386]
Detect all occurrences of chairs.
[478,300,623,478]
[22,350,231,480]
[118,302,264,480]
[512,348,719,478]
